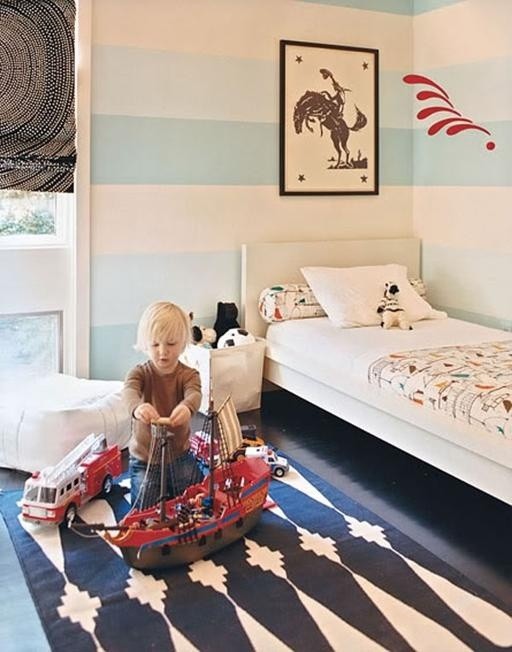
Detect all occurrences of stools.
[2,372,136,471]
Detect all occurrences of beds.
[237,234,512,505]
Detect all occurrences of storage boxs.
[179,333,266,417]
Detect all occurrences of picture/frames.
[278,37,381,196]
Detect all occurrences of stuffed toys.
[376,279,414,332]
[211,301,239,349]
[186,310,217,349]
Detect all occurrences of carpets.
[1,433,512,651]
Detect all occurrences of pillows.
[257,277,430,325]
[292,253,452,329]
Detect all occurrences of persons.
[126,299,204,514]
